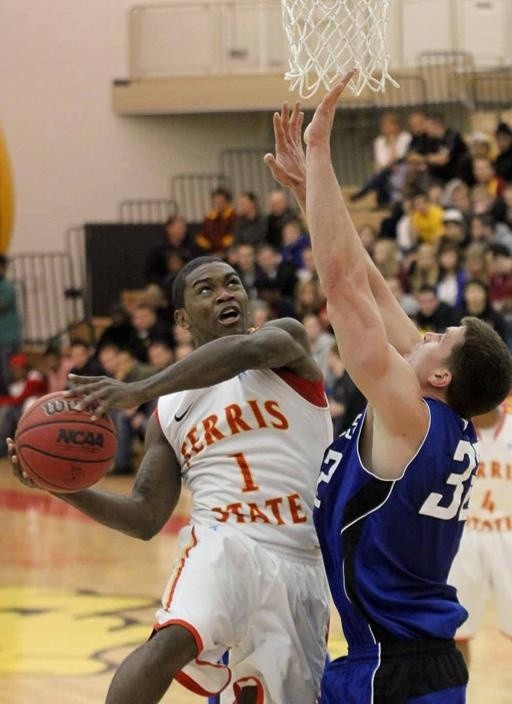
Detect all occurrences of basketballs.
[14,392,117,493]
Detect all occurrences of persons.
[264,67,512,704]
[7,257,334,703]
[0,108,512,475]
[446,404,512,665]
